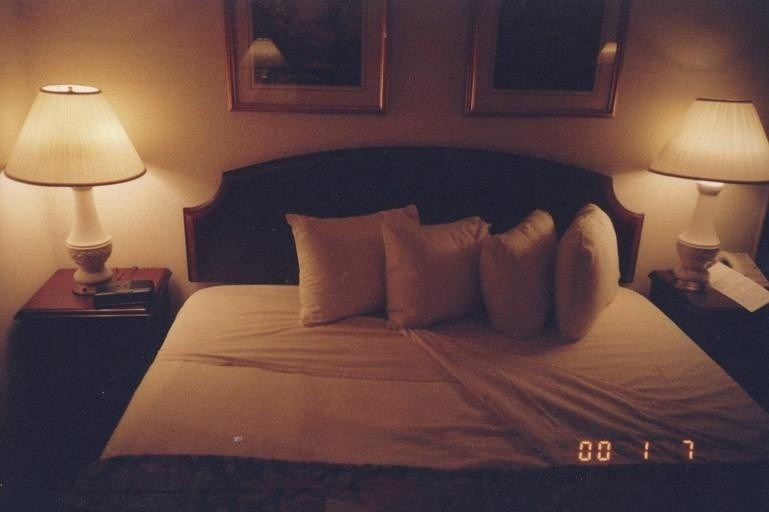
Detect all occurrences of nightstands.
[14,267,172,512]
[648,267,769,411]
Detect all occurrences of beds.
[71,145,768,512]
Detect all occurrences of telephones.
[715,250,769,289]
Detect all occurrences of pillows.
[556,201,621,344]
[286,201,420,326]
[379,207,491,330]
[478,209,556,342]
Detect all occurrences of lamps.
[4,84,147,297]
[647,97,769,292]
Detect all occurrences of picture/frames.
[223,0,393,115]
[464,0,631,118]
